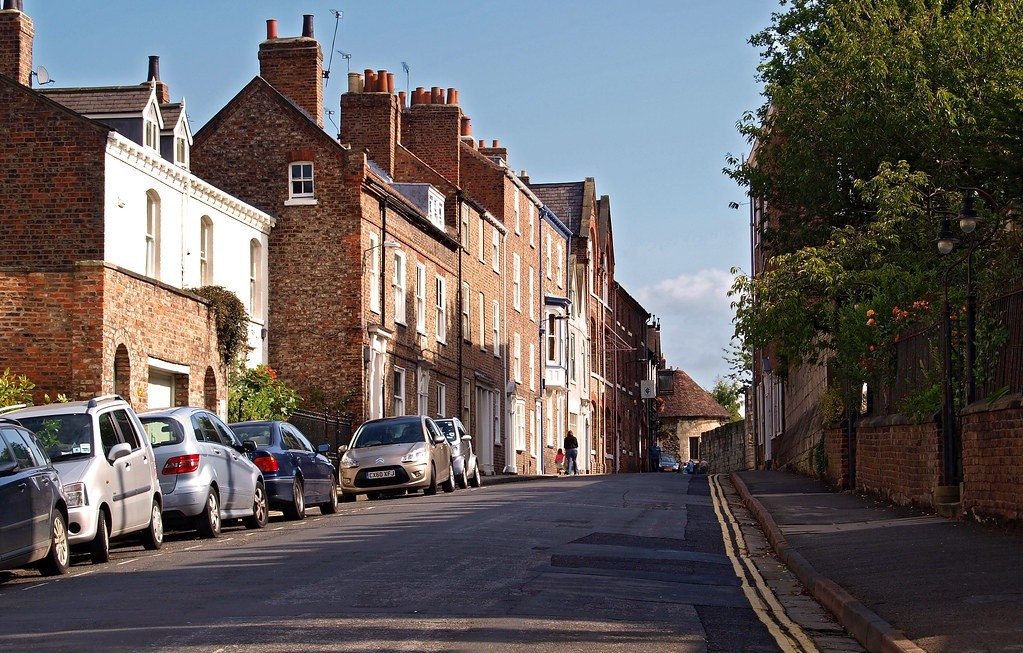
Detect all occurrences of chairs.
[58,418,88,448]
[400,428,421,440]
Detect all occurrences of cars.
[338,414,456,503]
[136,405,270,538]
[0,417,70,578]
[432,416,481,489]
[218,419,338,521]
[658,456,689,473]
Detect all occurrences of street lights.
[932,209,979,487]
[949,182,1003,405]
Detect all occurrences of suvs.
[0,393,164,565]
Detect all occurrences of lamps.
[363,241,401,267]
[542,315,570,322]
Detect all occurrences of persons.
[682,458,709,474]
[564,431,578,475]
[648,441,662,473]
[555,448,565,475]
